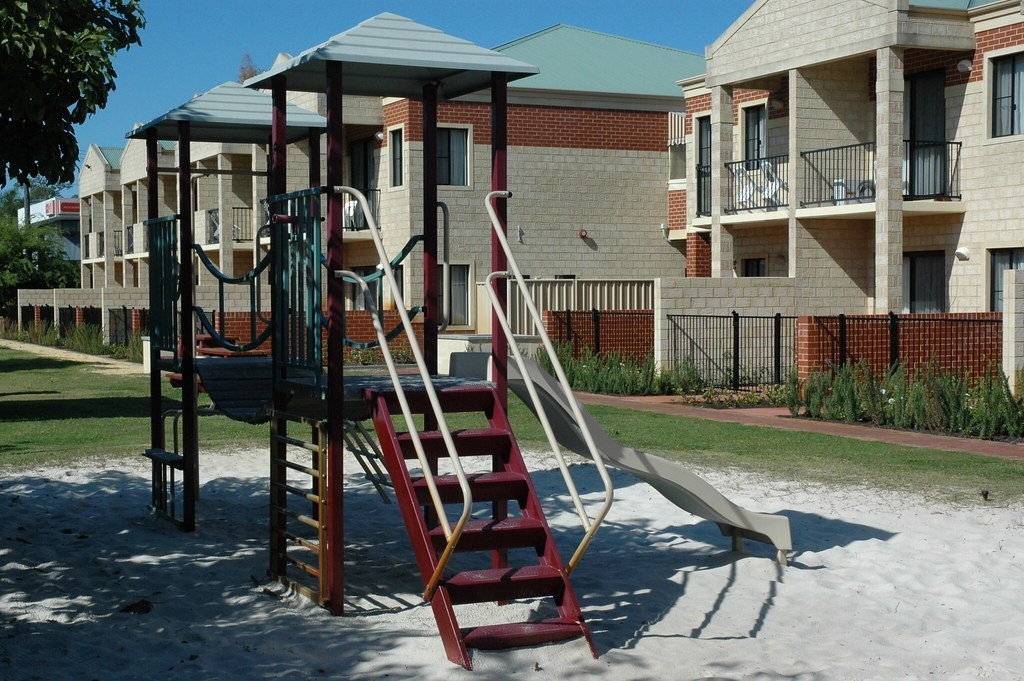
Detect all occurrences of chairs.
[735,166,765,213]
[211,212,241,241]
[761,161,788,206]
[343,200,358,231]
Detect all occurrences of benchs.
[165,373,205,392]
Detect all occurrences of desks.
[198,347,270,358]
[177,334,239,357]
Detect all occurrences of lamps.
[957,60,972,73]
[375,131,383,141]
[955,247,970,261]
[376,264,386,276]
